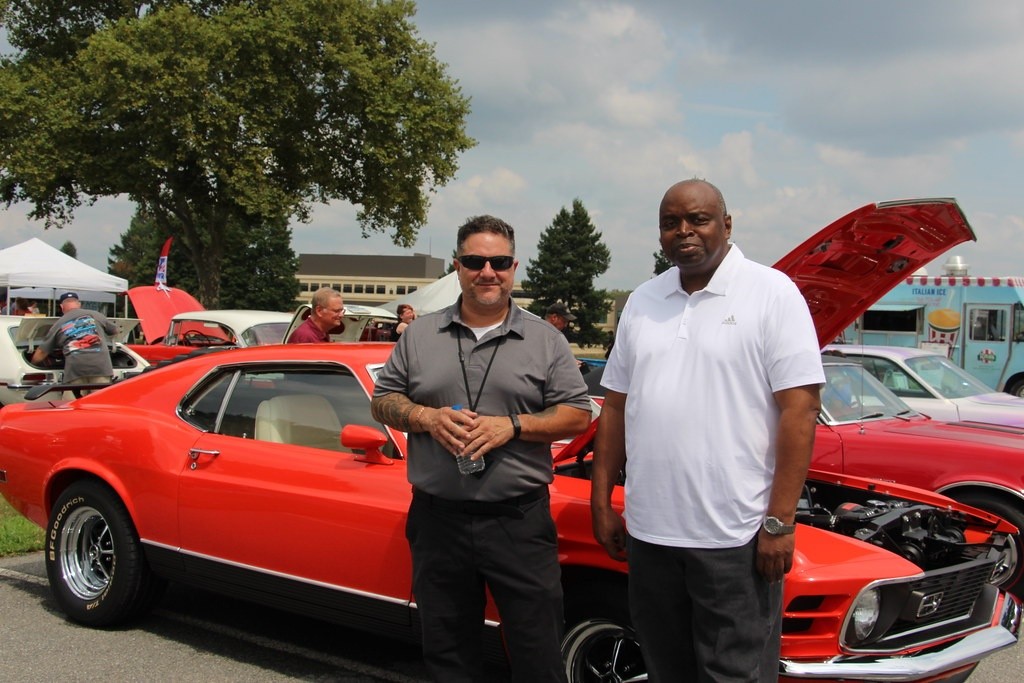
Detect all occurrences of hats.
[61,293,78,303]
[546,302,577,321]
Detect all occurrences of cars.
[820,344,1024,433]
[1,313,150,408]
[1,197,1018,677]
[162,302,398,345]
[118,285,231,367]
[806,359,1023,585]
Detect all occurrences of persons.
[287,287,344,344]
[2,297,39,315]
[30,292,120,400]
[389,304,417,343]
[604,331,615,359]
[371,215,594,683]
[589,178,826,683]
[545,302,577,332]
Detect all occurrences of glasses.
[457,255,514,271]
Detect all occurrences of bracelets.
[509,413,521,439]
[415,406,426,432]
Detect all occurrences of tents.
[9,286,116,317]
[0,238,128,318]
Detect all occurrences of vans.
[831,277,1022,397]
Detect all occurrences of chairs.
[255,395,353,454]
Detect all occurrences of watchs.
[764,516,796,535]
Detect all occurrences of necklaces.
[456,325,502,414]
